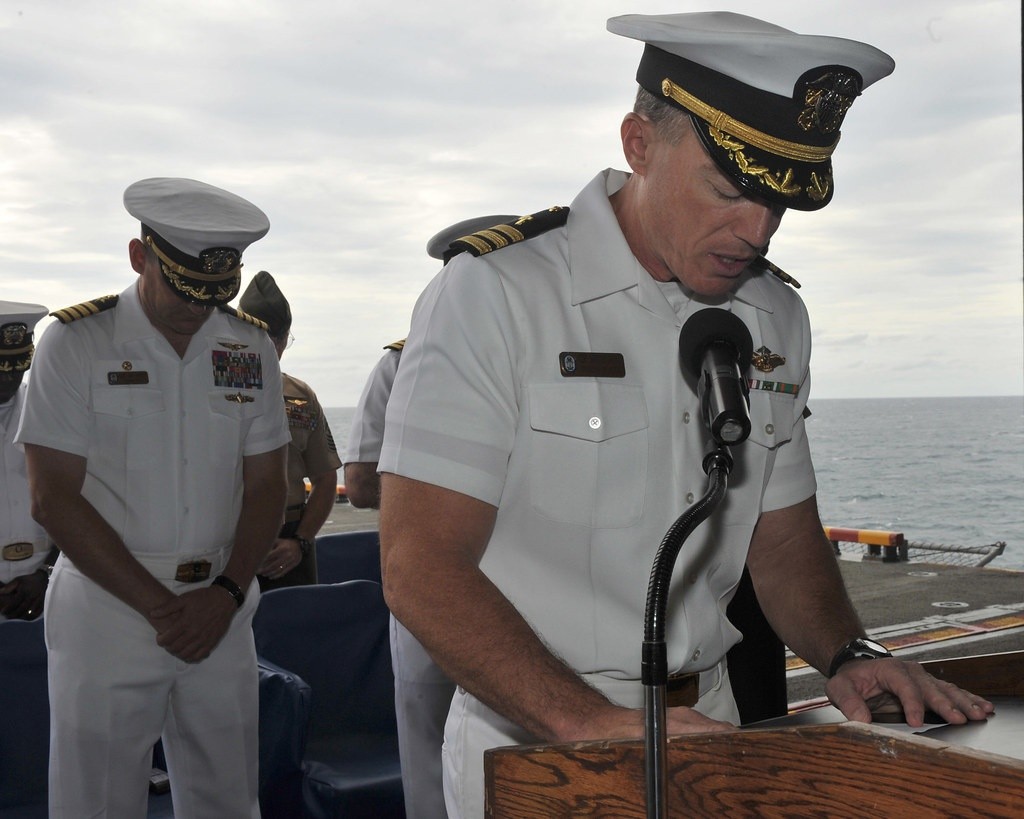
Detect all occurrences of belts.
[578,660,731,707]
[3,539,48,561]
[135,547,236,582]
[282,508,304,525]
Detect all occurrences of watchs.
[827,637,892,679]
[37,562,54,578]
[283,533,313,558]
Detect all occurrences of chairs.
[0,530,406,819]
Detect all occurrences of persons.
[1,300,61,621]
[12,177,292,819]
[344,213,522,819]
[236,271,342,594]
[376,11,995,819]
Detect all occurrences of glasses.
[270,331,295,351]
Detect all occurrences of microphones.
[679,308,754,445]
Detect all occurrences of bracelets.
[211,575,245,609]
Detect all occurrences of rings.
[280,564,285,571]
[28,609,36,617]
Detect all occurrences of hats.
[239,272,292,336]
[605,11,895,211]
[0,299,49,373]
[426,214,521,261]
[123,176,270,304]
